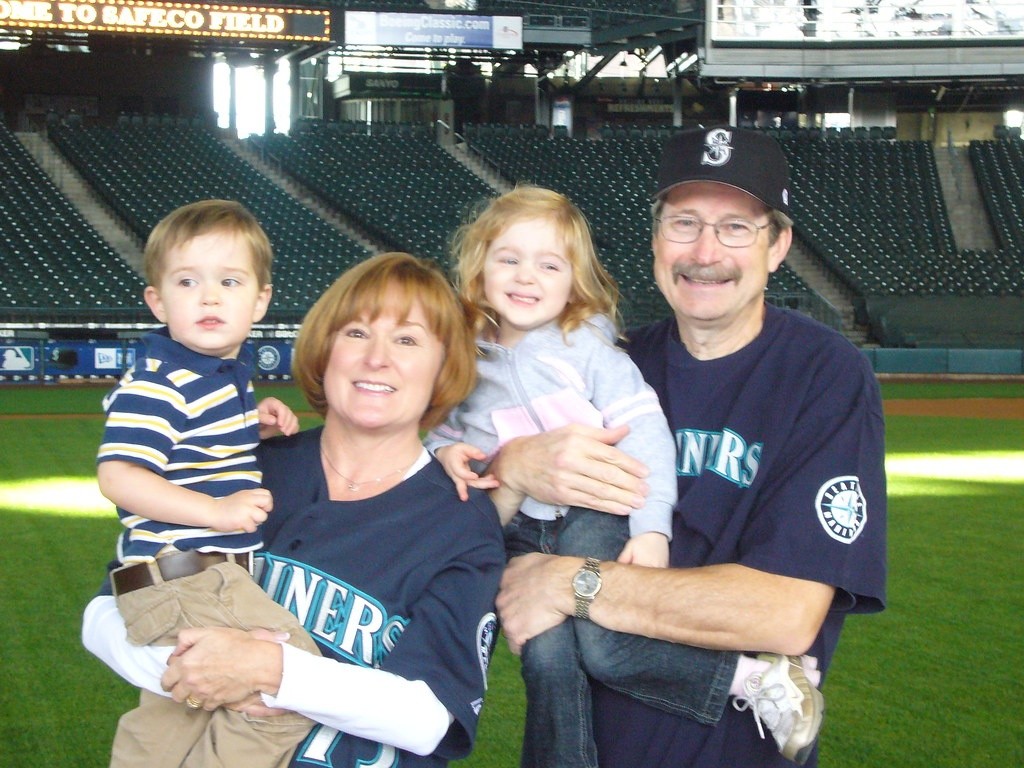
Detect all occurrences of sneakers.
[739,655,824,767]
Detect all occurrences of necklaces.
[319,447,411,491]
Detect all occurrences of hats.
[651,124,794,228]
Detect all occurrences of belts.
[114,549,249,595]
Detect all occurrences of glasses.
[655,216,771,248]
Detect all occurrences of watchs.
[572,557,602,618]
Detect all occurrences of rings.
[187,696,202,708]
[501,631,506,638]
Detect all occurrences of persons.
[480,128,886,768]
[95,198,324,768]
[422,185,826,768]
[83,253,506,768]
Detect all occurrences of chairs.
[0,113,1024,322]
